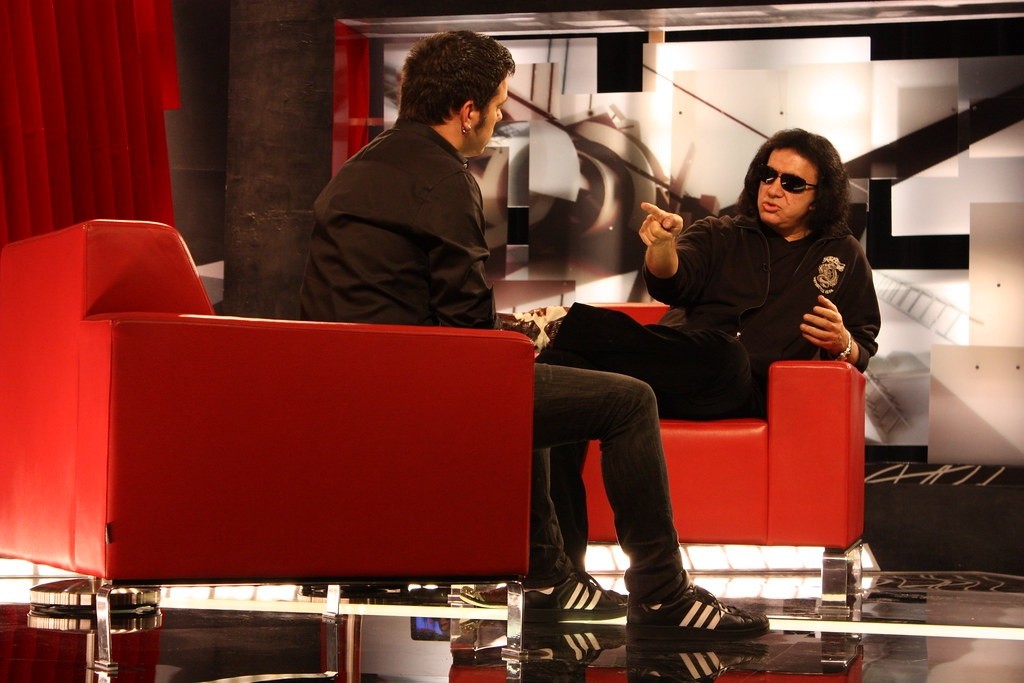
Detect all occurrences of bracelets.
[835,332,852,360]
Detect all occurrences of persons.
[458,127,882,610]
[515,621,768,682]
[301,27,769,643]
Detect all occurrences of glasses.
[753,163,819,193]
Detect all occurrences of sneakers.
[624,644,769,683]
[623,583,770,641]
[522,624,627,682]
[524,568,628,622]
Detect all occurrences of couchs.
[0,219,535,683]
[537,302,866,606]
